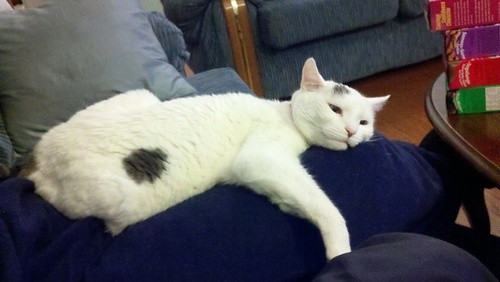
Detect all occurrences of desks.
[418,69,500,248]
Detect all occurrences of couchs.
[0,11,493,282]
[160,0,445,99]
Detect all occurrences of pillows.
[0,0,184,153]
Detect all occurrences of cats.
[16,57,391,262]
